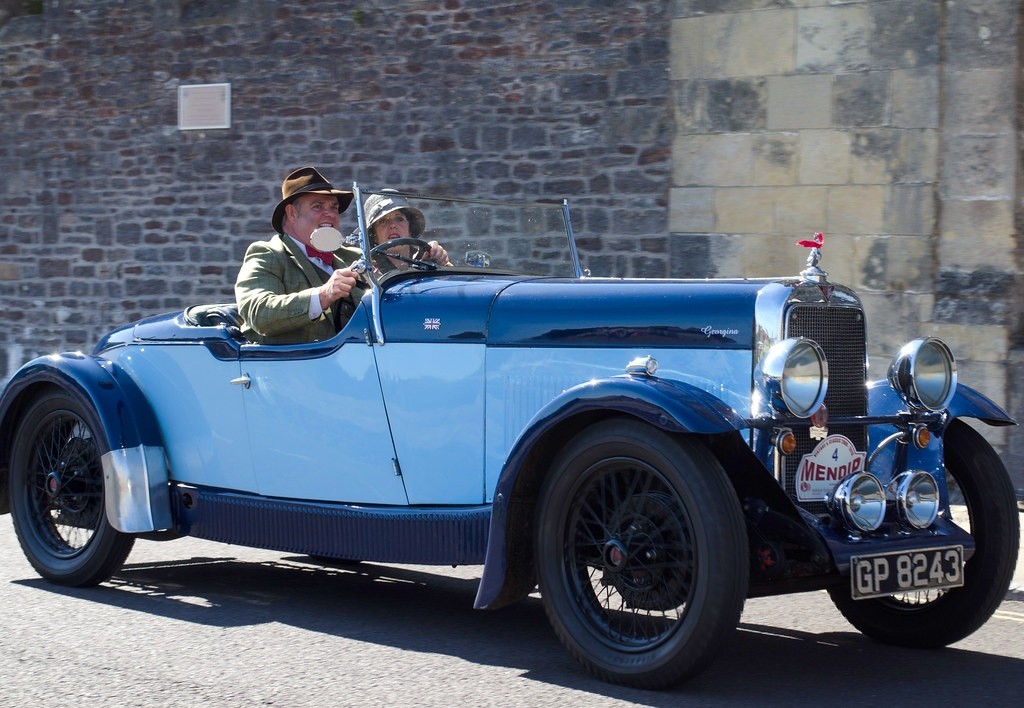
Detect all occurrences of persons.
[234,166,450,345]
[346,188,426,275]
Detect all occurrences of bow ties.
[305,244,335,265]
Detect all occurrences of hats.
[363,189,426,239]
[271,166,354,234]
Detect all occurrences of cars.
[0,174,1024,690]
